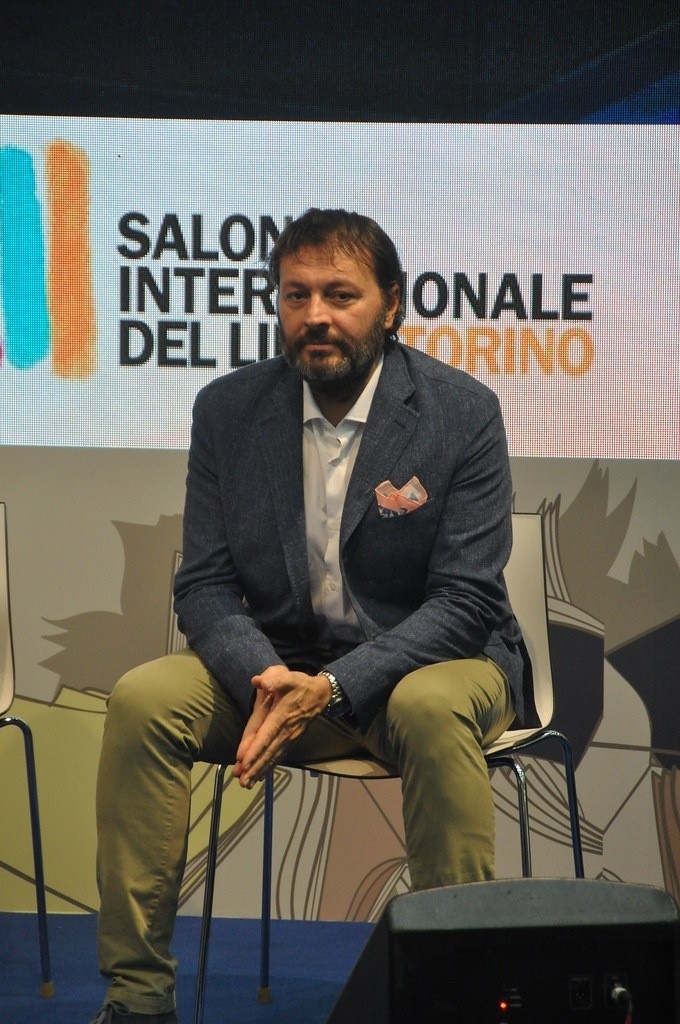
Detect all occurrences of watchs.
[318,671,351,718]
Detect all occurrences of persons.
[89,208,542,1024]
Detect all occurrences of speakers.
[323,877,680,1024]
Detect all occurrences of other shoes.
[87,1000,178,1024]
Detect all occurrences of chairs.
[0,494,56,1001]
[191,509,585,1023]
